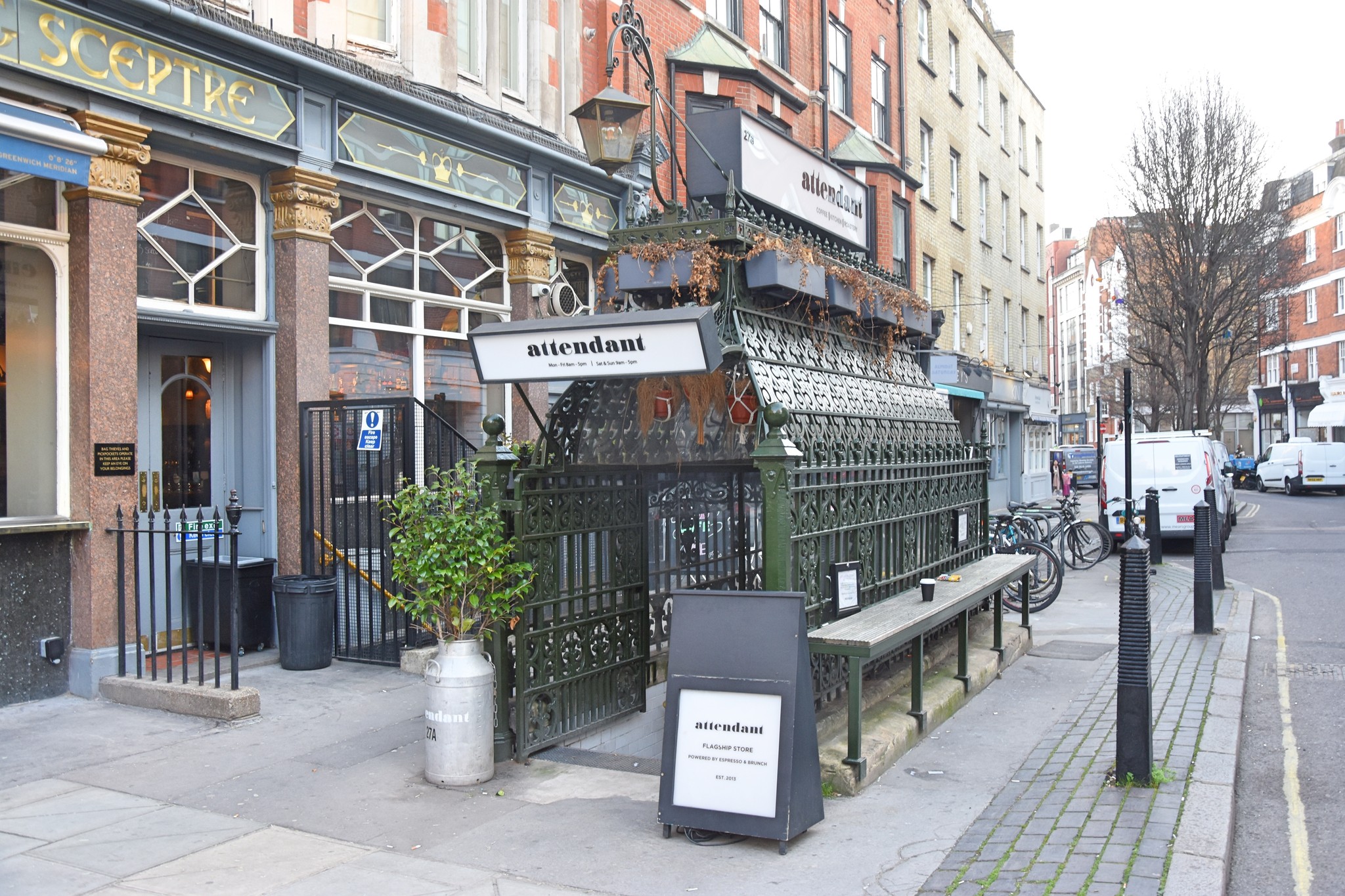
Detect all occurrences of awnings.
[1307,401,1345,428]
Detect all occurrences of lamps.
[1013,370,1032,378]
[186,385,199,400]
[1032,376,1048,382]
[1040,380,1061,387]
[957,356,971,377]
[969,357,982,377]
[202,358,211,373]
[991,365,1014,373]
[974,360,991,380]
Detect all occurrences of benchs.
[807,554,1037,782]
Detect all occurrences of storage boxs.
[1235,458,1254,470]
[186,556,277,657]
[1230,459,1236,465]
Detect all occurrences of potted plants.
[637,376,682,439]
[727,379,758,425]
[376,458,540,785]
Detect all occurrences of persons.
[1050,459,1061,496]
[1234,445,1242,458]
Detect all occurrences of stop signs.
[1099,423,1106,434]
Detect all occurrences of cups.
[920,578,936,601]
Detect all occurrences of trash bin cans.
[181,554,277,656]
[271,574,337,671]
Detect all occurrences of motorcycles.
[1241,461,1260,490]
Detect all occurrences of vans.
[1050,448,1098,488]
[1255,441,1345,496]
[1098,428,1237,553]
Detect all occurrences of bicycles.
[972,486,1114,618]
[1105,492,1161,577]
